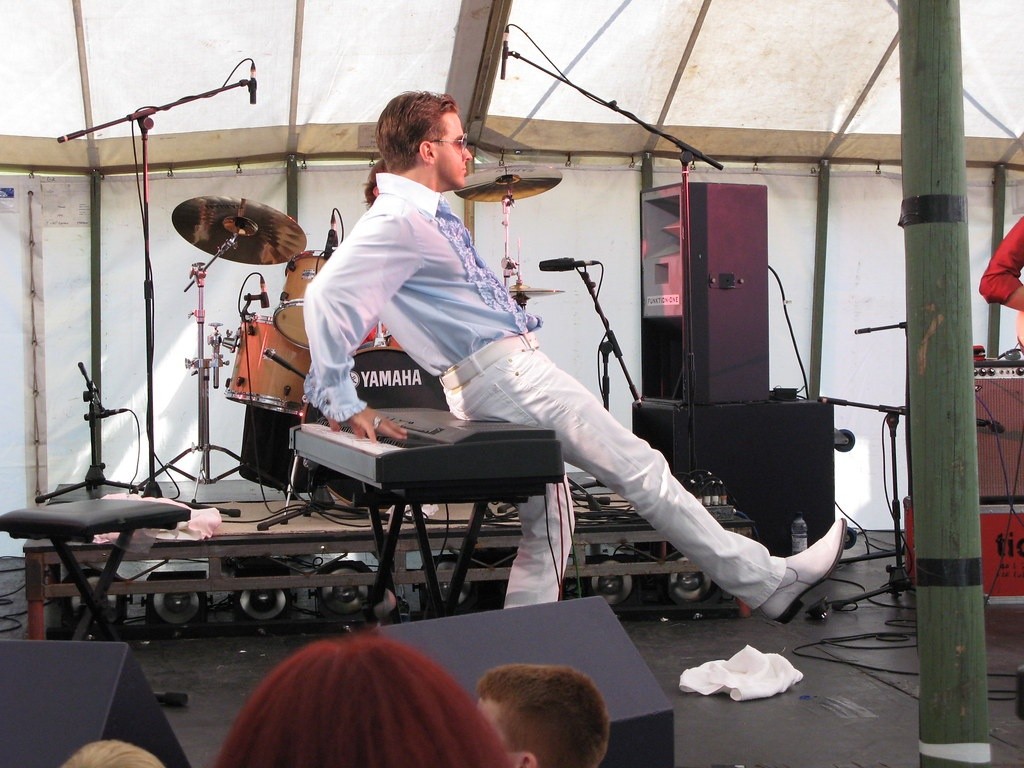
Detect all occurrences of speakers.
[971,355,1024,506]
[0,638,192,768]
[633,399,836,558]
[639,182,770,405]
[371,595,676,768]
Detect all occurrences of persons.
[59,633,611,768]
[310,158,404,509]
[301,92,848,625]
[980,216,1024,355]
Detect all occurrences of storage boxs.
[902,495,1024,604]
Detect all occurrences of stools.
[0,498,189,708]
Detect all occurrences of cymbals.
[171,194,307,264]
[455,161,564,207]
[508,282,567,302]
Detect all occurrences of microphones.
[987,420,1004,434]
[539,258,600,272]
[330,214,338,252]
[259,276,269,308]
[249,64,257,104]
[501,27,509,80]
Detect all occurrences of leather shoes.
[761,517,849,625]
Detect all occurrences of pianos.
[285,404,567,625]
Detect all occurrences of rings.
[373,415,384,432]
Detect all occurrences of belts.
[439,331,540,388]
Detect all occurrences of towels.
[679,645,804,703]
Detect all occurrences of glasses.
[417,125,468,152]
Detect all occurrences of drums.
[273,250,328,351]
[299,347,448,510]
[225,314,313,415]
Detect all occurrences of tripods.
[35,77,415,532]
[816,396,991,610]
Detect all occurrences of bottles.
[791,511,808,555]
